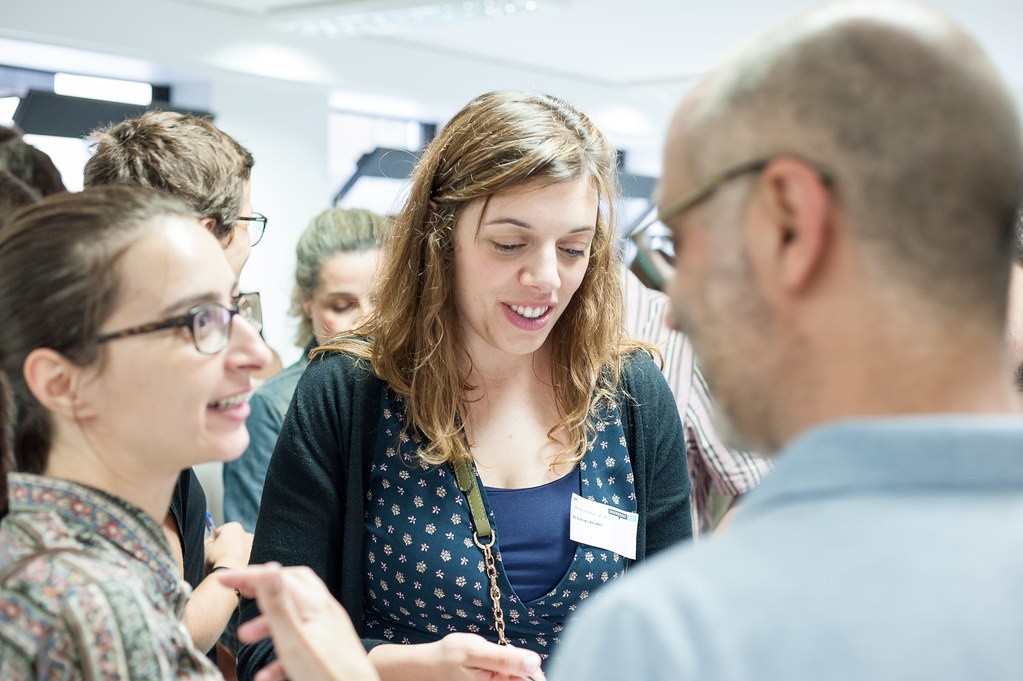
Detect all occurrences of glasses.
[226,210,266,246]
[56,293,264,355]
[619,154,835,294]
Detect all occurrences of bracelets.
[208,566,241,599]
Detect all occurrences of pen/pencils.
[206,513,219,537]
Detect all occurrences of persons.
[618,260,774,542]
[0,130,378,681]
[234,90,695,681]
[547,0,1023,681]
[222,208,393,646]
[84,114,255,666]
